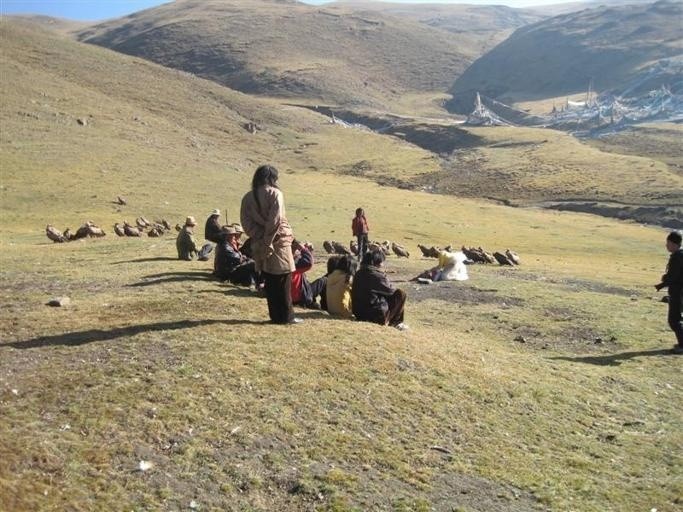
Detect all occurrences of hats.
[667,231,682,244]
[221,223,245,234]
[211,209,221,216]
[184,216,197,225]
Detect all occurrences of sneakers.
[279,315,303,325]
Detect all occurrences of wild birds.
[323,238,358,258]
[45,216,181,244]
[416,244,520,266]
[369,239,409,258]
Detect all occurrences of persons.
[204,208,223,243]
[238,165,305,325]
[214,222,265,291]
[175,215,213,262]
[350,207,370,263]
[291,238,409,330]
[654,231,683,355]
[429,246,457,280]
